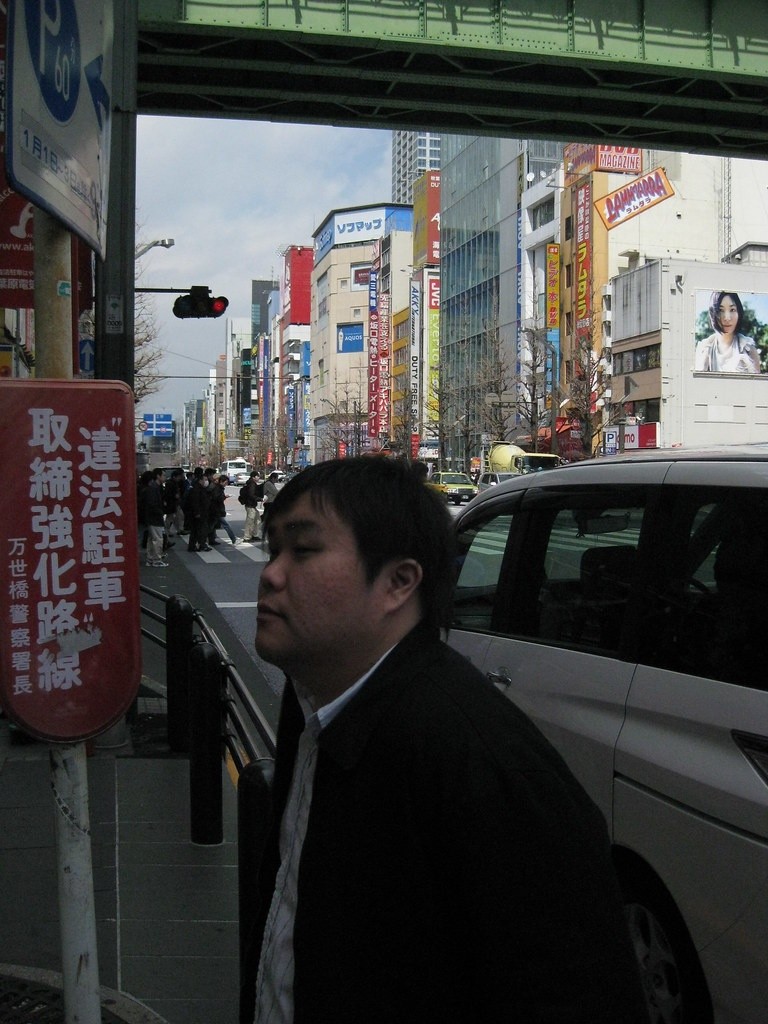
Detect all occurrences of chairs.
[696,535,768,687]
[547,543,669,660]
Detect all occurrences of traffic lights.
[172,295,229,320]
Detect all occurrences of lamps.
[546,177,567,190]
[565,163,590,176]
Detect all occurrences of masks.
[203,481,209,486]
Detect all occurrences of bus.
[221,460,247,486]
[181,465,189,473]
[136,450,151,478]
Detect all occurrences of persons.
[133,466,278,568]
[227,454,722,1024]
[692,290,763,374]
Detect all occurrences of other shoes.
[243,539,252,543]
[188,548,200,552]
[145,559,153,566]
[199,546,211,551]
[253,537,262,542]
[232,538,236,544]
[167,541,176,547]
[152,560,170,567]
[209,542,221,545]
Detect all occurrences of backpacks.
[238,484,248,505]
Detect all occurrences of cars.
[424,471,479,506]
[478,473,522,494]
[270,471,286,482]
[436,448,767,1024]
[154,466,181,482]
[234,472,251,487]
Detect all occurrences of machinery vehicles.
[481,440,560,476]
